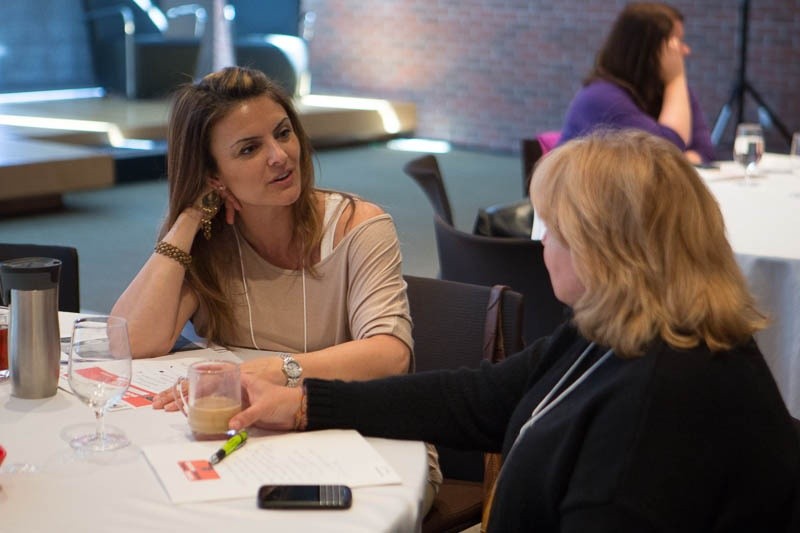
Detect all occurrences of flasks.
[0,257,62,399]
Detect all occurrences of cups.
[0,306,10,377]
[789,133,800,177]
[172,360,243,441]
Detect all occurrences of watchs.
[279,351,303,388]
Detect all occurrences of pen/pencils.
[696,165,720,169]
[208,431,248,464]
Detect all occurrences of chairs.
[406,127,574,532]
[0,243,81,313]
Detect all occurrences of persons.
[108,65,418,414]
[554,3,716,174]
[190,126,800,533]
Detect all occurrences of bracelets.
[152,240,193,272]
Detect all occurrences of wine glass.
[733,123,765,184]
[68,316,132,453]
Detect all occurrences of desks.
[0,307,441,533]
[693,150,799,420]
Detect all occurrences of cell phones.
[258,485,352,510]
